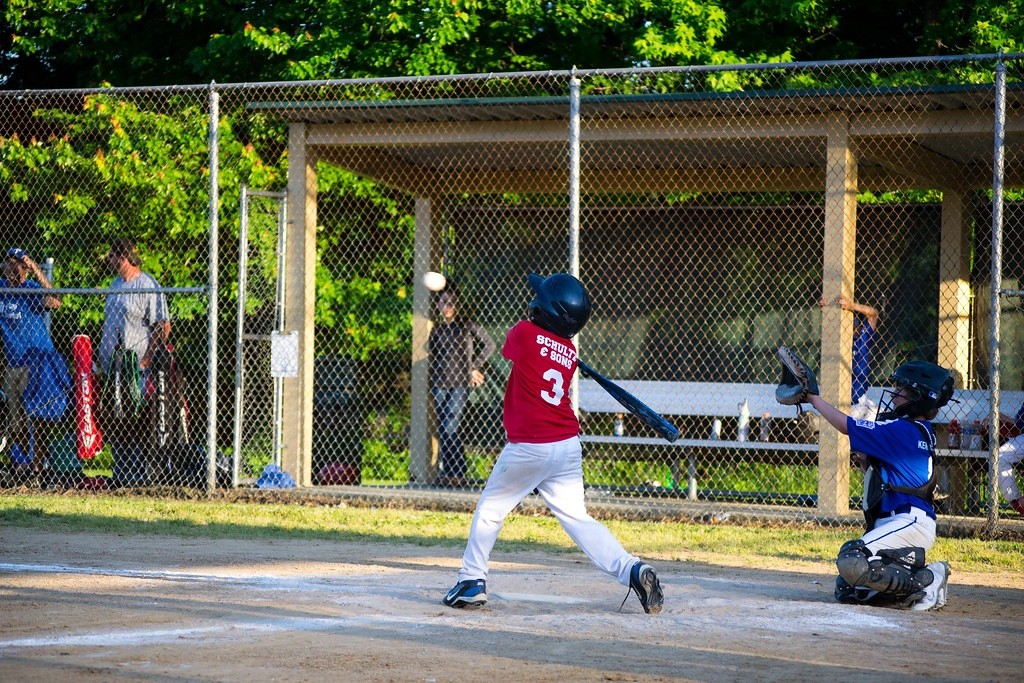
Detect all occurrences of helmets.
[528,273,592,339]
[875,361,955,421]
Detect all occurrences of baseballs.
[424,272,447,291]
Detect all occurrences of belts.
[877,506,929,518]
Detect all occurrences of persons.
[429,279,497,487]
[984,401,1024,518]
[820,299,878,471]
[775,344,955,613]
[0,239,170,487]
[444,273,664,615]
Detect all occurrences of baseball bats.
[576,358,680,443]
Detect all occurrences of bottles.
[614,414,624,436]
[946,418,961,450]
[961,418,971,450]
[738,398,749,443]
[969,420,983,451]
[711,418,722,440]
[761,413,771,442]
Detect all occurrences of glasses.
[109,253,115,258]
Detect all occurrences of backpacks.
[145,342,190,449]
[17,347,76,421]
[99,346,145,421]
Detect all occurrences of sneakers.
[442,579,488,608]
[911,560,951,612]
[618,561,665,614]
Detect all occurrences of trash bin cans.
[311,354,363,485]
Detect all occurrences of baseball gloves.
[776,345,820,406]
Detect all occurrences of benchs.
[579,380,1024,516]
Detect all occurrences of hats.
[7,248,28,262]
[112,237,140,265]
